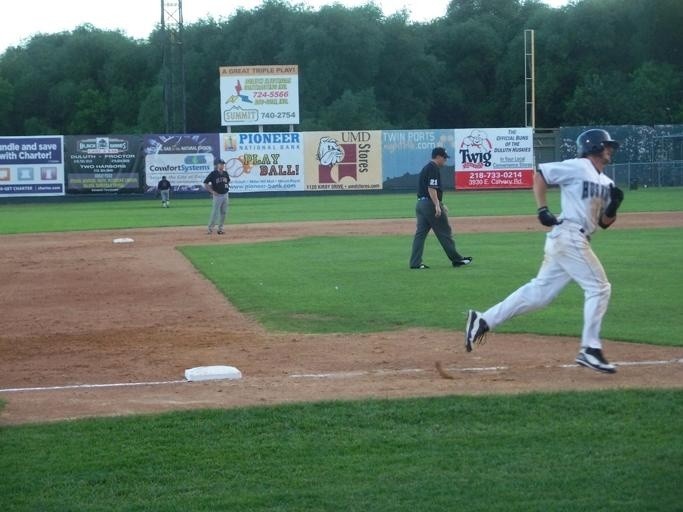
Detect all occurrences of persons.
[462,127,624,374]
[202,157,232,236]
[406,147,473,270]
[156,175,171,209]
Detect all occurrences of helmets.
[576,128,619,157]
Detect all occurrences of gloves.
[609,183,624,207]
[537,206,557,226]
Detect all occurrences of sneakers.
[575,347,617,373]
[464,309,489,352]
[453,257,472,267]
[410,264,430,269]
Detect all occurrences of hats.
[433,147,450,158]
[216,159,225,164]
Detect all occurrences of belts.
[417,196,430,201]
